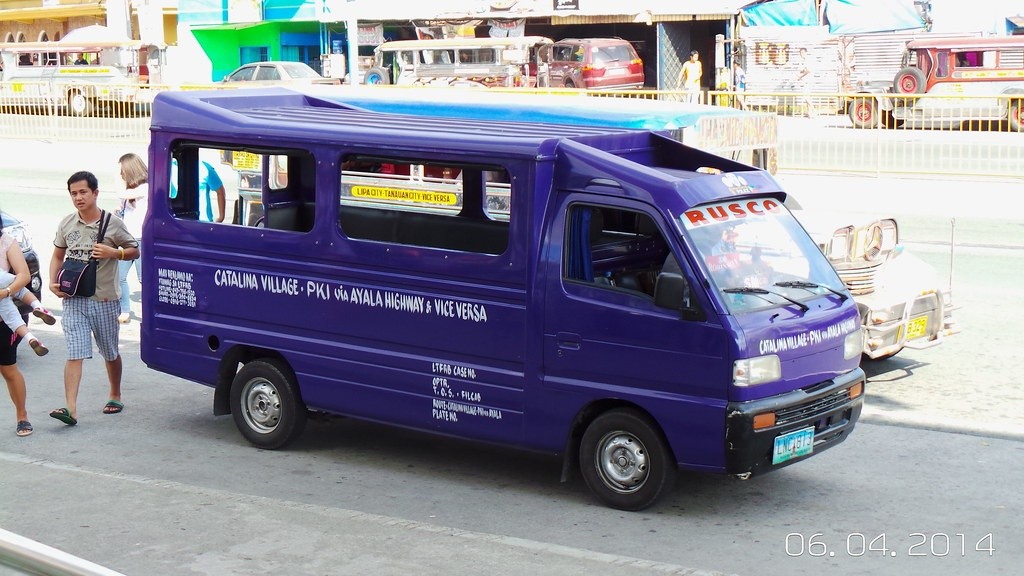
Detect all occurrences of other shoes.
[118,313,132,324]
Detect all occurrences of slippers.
[15,420,35,437]
[49,407,78,426]
[102,399,125,414]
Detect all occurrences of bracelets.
[120,249,125,260]
[6,288,11,297]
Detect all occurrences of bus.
[0,40,168,117]
[837,36,1024,131]
[363,36,554,88]
[233,89,966,360]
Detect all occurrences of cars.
[178,60,341,90]
[549,37,644,89]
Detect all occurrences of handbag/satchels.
[55,209,111,298]
[8,246,43,315]
[113,198,127,220]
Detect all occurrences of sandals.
[32,306,56,326]
[28,338,49,356]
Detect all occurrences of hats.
[19,56,26,59]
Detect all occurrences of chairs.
[609,271,653,295]
[18,55,34,65]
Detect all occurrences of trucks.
[139,88,867,512]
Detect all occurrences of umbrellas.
[61,24,131,42]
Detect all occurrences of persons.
[74,52,88,65]
[678,50,702,103]
[732,60,749,111]
[111,152,149,320]
[0,268,56,356]
[169,156,226,223]
[797,48,817,119]
[0,215,33,436]
[49,171,140,425]
